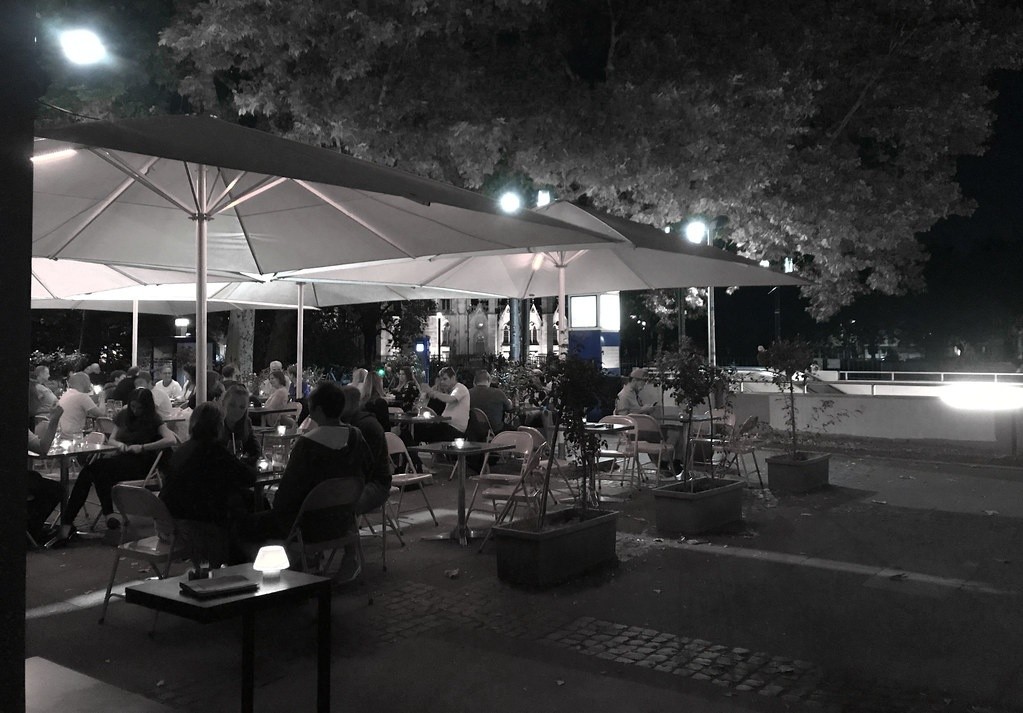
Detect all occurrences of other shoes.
[53,525,78,548]
[336,560,361,583]
[25,527,42,548]
[107,518,121,529]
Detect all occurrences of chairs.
[30,380,767,572]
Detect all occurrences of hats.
[630,368,649,379]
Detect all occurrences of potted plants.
[638,335,748,539]
[755,335,866,493]
[481,333,623,591]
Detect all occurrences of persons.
[28,352,512,584]
[615,368,682,478]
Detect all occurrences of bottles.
[273,459,284,479]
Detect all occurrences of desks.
[247,405,298,417]
[85,411,186,422]
[389,414,453,487]
[655,413,724,484]
[125,562,333,713]
[27,438,121,551]
[405,440,517,548]
[252,426,308,464]
[250,457,285,486]
[546,421,636,508]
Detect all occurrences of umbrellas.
[32,108,819,427]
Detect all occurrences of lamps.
[252,545,290,578]
[174,317,191,338]
[277,425,287,437]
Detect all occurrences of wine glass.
[50,426,64,454]
[73,431,82,451]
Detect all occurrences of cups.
[195,567,214,580]
[265,447,273,460]
[107,400,122,419]
[273,445,285,463]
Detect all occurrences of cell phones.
[653,402,658,405]
